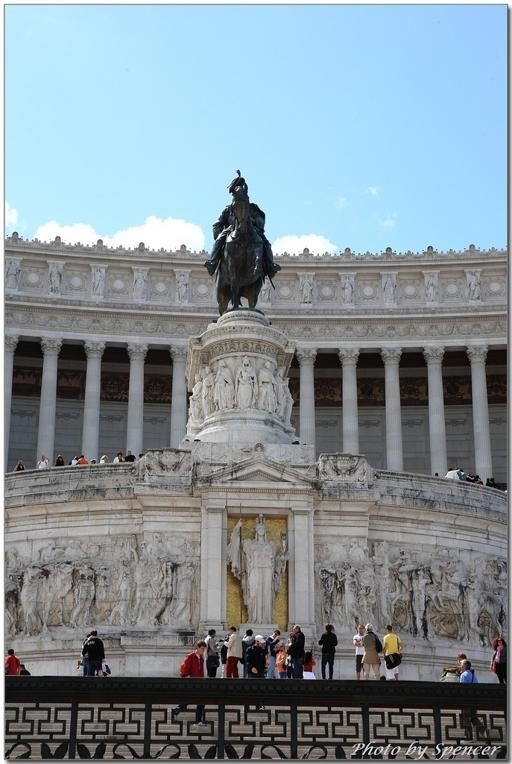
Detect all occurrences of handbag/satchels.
[102,664,112,676]
[384,652,403,669]
[208,655,220,668]
[372,632,383,653]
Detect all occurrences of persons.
[13,450,143,472]
[204,177,281,278]
[434,467,497,488]
[189,355,295,425]
